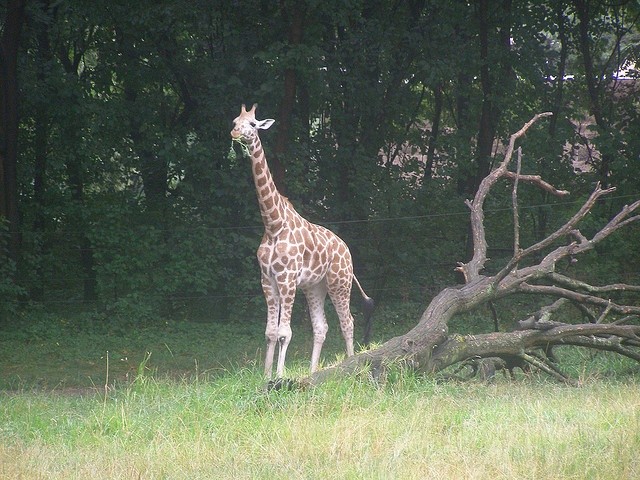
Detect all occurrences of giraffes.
[230,103,374,382]
